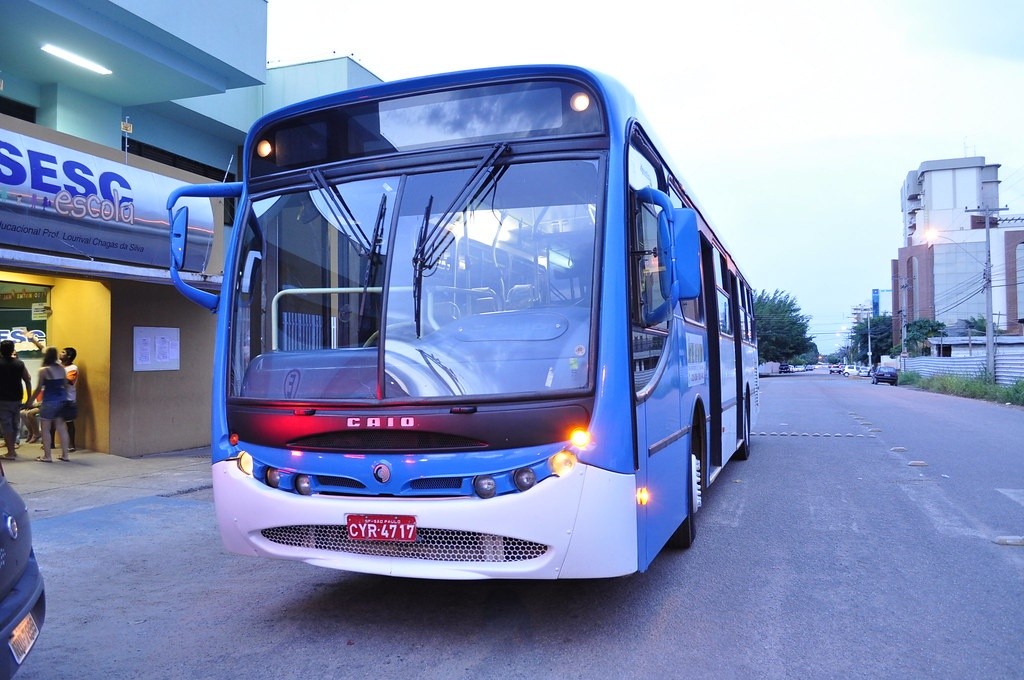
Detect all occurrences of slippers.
[37,455,52,462]
[57,454,69,461]
[0,453,16,460]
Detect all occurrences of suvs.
[828,363,874,377]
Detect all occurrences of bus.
[166,64,759,580]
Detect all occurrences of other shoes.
[29,436,41,443]
[68,445,76,452]
[0,442,6,447]
[41,443,54,449]
[14,443,20,449]
[26,434,33,442]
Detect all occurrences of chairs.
[468,286,503,312]
[507,282,543,310]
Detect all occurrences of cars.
[871,366,898,387]
[778,364,814,374]
[0,459,46,680]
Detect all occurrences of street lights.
[924,233,994,387]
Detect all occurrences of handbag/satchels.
[61,402,78,421]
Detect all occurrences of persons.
[0,350,21,448]
[41,347,79,452]
[19,388,44,443]
[0,340,32,460]
[26,347,70,462]
